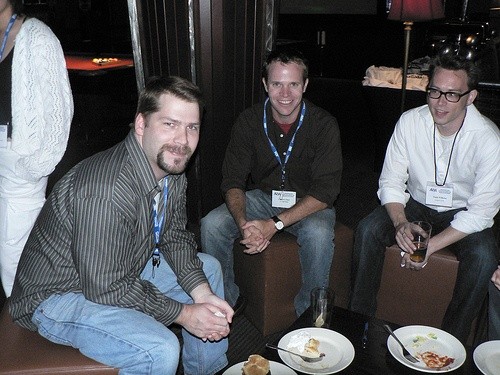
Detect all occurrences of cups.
[310,286,336,329]
[409,221,432,263]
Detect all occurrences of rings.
[402,232,407,239]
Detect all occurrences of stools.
[373,241,477,346]
[234,219,353,337]
[0,297,120,375]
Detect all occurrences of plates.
[386,325,467,373]
[473,340,500,375]
[222,360,297,375]
[277,327,355,375]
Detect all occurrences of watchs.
[271,216,284,232]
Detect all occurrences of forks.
[383,324,420,364]
[266,343,322,363]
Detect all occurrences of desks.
[260,304,484,375]
[64,50,136,145]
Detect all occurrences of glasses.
[425,82,471,103]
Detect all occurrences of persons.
[0,0,73,298]
[201,45,343,319]
[348,52,500,349]
[9,75,235,375]
[488,264,500,340]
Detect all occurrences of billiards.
[92,57,118,63]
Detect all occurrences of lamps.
[388,0,445,117]
